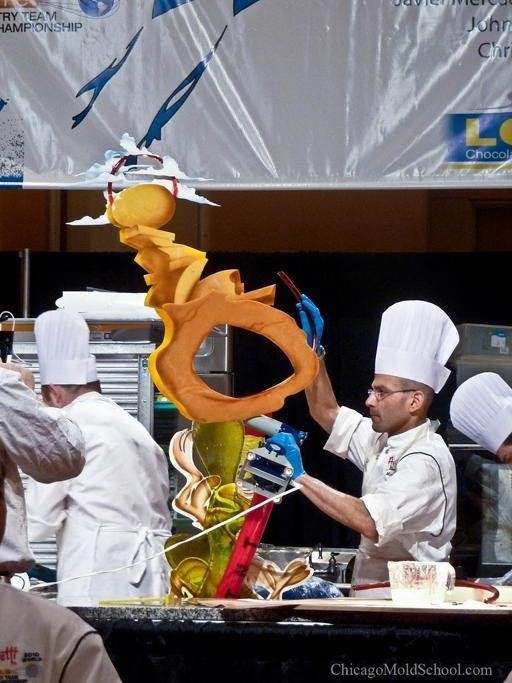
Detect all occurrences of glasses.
[367,386,417,402]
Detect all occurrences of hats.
[34,310,98,386]
[373,298,461,394]
[449,371,512,456]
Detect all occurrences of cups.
[387,559,456,607]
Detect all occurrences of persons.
[266,293,457,597]
[0,456,125,682]
[0,351,87,594]
[23,366,174,609]
[490,423,511,471]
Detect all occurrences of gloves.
[269,432,307,480]
[296,293,328,360]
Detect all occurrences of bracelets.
[317,344,327,359]
